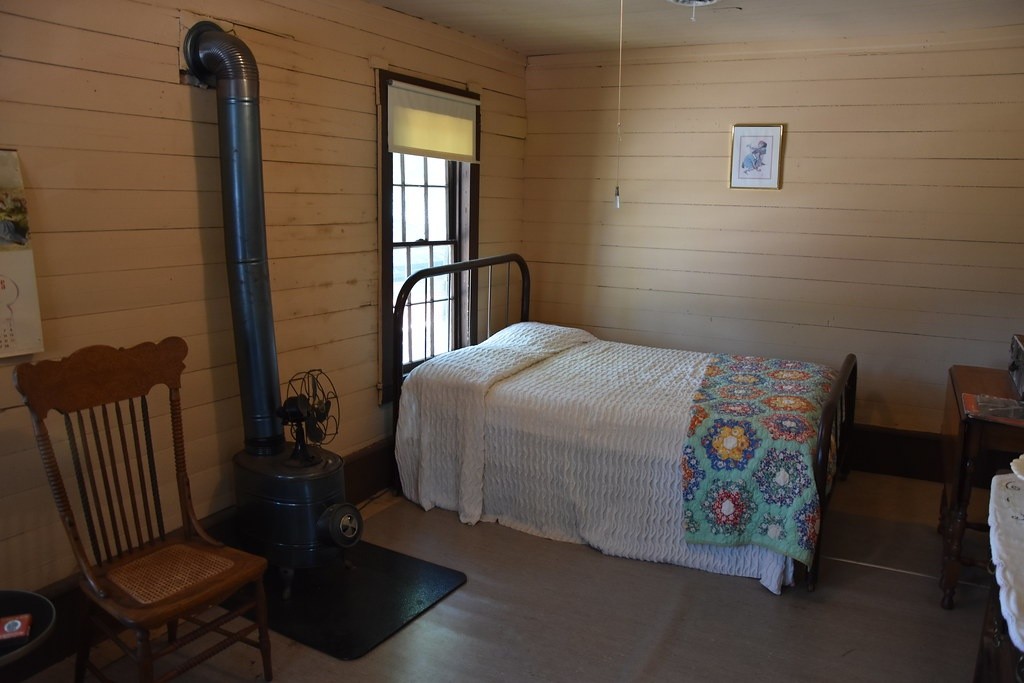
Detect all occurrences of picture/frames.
[727,122,785,191]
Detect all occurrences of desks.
[938,334,1024,609]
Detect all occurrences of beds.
[389,251,859,593]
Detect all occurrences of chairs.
[12,336,274,683]
